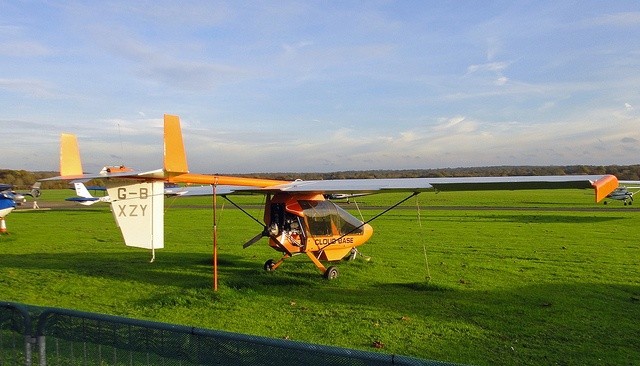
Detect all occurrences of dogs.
[268,222,279,236]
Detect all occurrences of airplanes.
[39,114,618,290]
[0,183,26,217]
[604,186,633,205]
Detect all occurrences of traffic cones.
[0,219,7,232]
